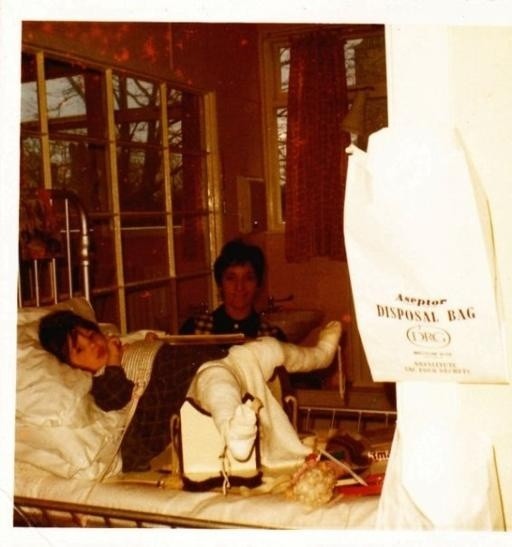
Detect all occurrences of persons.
[179,237,289,344]
[38,306,343,473]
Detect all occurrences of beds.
[16,188,398,526]
